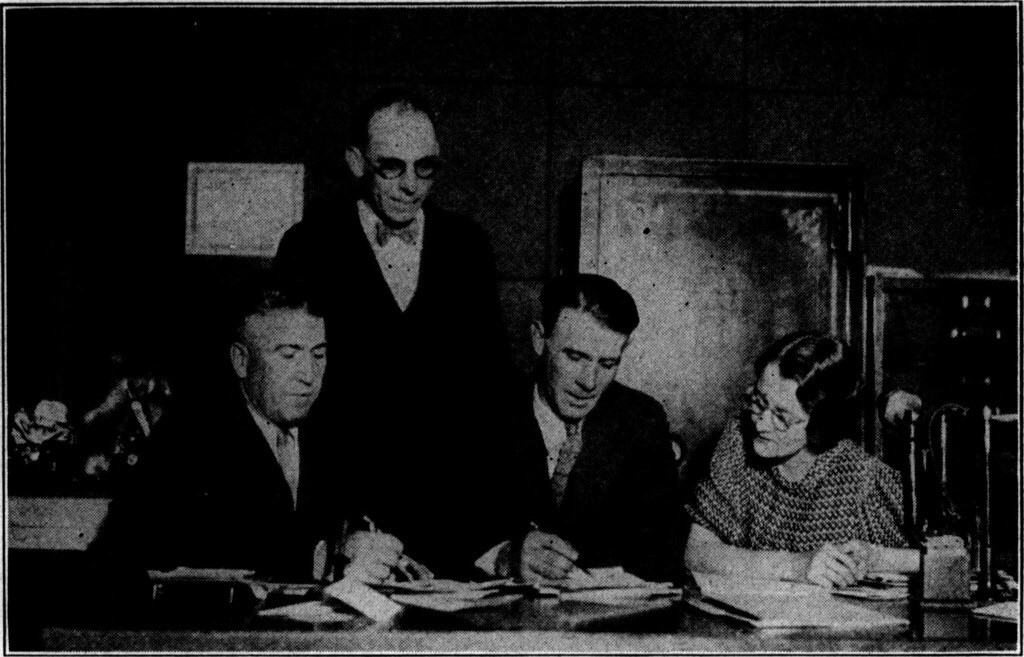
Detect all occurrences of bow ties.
[375,222,418,248]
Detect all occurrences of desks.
[42,578,1020,654]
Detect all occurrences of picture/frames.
[185,161,307,259]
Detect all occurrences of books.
[685,568,908,629]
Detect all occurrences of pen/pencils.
[361,514,421,582]
[530,520,594,577]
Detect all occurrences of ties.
[551,423,582,507]
[276,429,297,509]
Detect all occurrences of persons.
[277,101,534,574]
[684,319,923,588]
[421,272,693,591]
[64,272,403,630]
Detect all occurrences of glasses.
[361,152,447,181]
[748,387,811,433]
